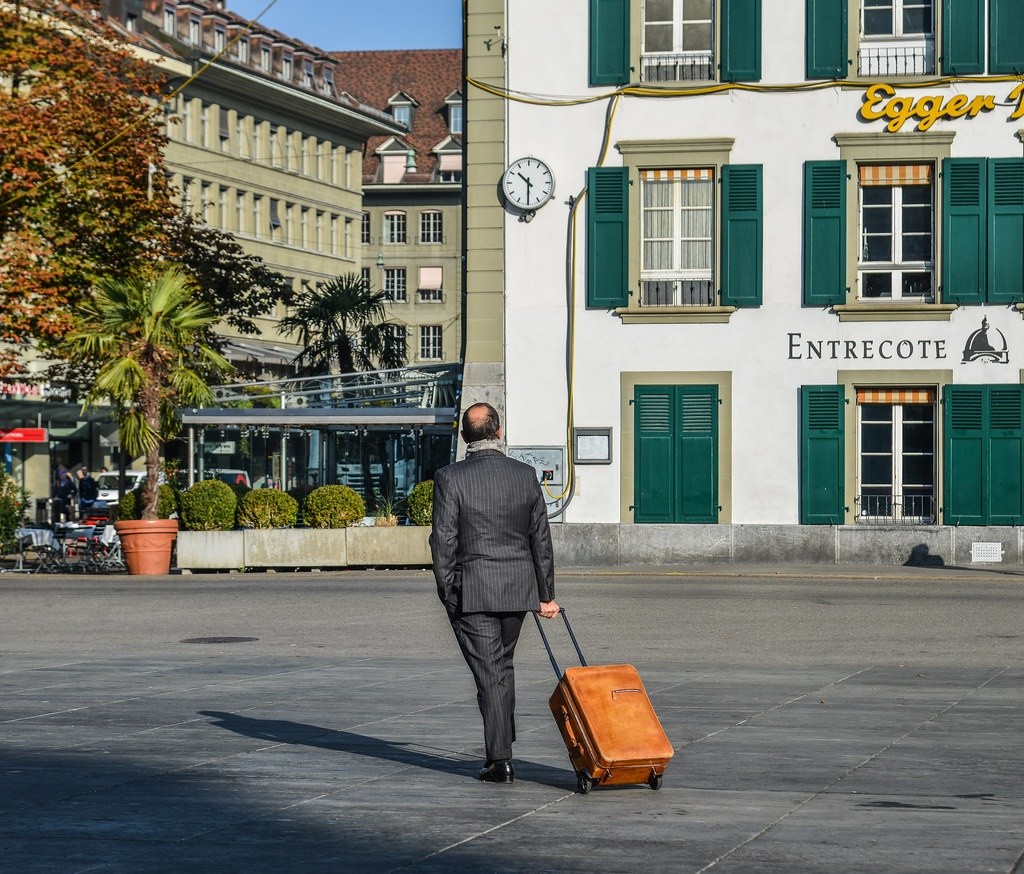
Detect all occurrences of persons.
[51,466,109,522]
[428,402,558,784]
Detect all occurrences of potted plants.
[177,478,436,573]
[62,262,237,575]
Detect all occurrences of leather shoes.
[484,759,494,767]
[479,760,514,783]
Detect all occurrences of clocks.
[503,157,555,223]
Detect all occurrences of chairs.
[31,524,127,574]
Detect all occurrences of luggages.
[533,607,675,793]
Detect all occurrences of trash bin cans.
[36,497,48,523]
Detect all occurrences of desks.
[1,528,62,573]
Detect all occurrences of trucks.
[335,432,421,500]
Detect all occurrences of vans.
[95,468,252,505]
